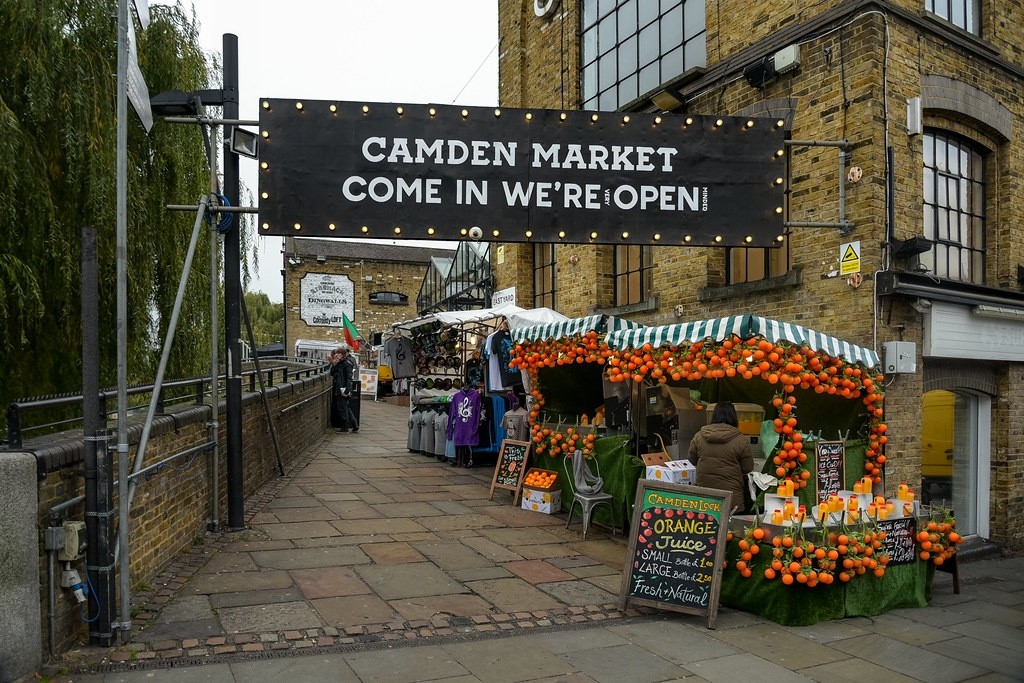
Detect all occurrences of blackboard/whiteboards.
[491,438,531,491]
[815,439,846,508]
[618,477,734,616]
[876,515,917,567]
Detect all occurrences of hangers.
[462,382,470,393]
[496,320,509,332]
[392,327,403,338]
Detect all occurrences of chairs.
[563,452,615,541]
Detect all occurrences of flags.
[342,312,364,352]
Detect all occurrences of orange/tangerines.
[723,519,963,587]
[524,471,556,488]
[508,331,612,460]
[606,337,885,492]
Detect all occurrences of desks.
[719,514,934,625]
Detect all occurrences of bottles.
[770,509,783,525]
[800,505,806,516]
[776,484,787,497]
[818,475,915,524]
[798,508,806,523]
[783,477,794,496]
[783,500,795,520]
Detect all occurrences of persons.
[327,347,360,435]
[688,401,754,515]
[500,403,529,442]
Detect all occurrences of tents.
[390,309,880,479]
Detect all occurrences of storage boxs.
[641,452,696,486]
[520,467,560,493]
[521,486,562,515]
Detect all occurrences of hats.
[407,319,464,390]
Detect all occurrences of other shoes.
[352,427,360,433]
[335,427,350,435]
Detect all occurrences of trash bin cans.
[332,380,361,429]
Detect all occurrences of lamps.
[648,86,685,111]
[222,125,258,160]
[970,304,1024,321]
